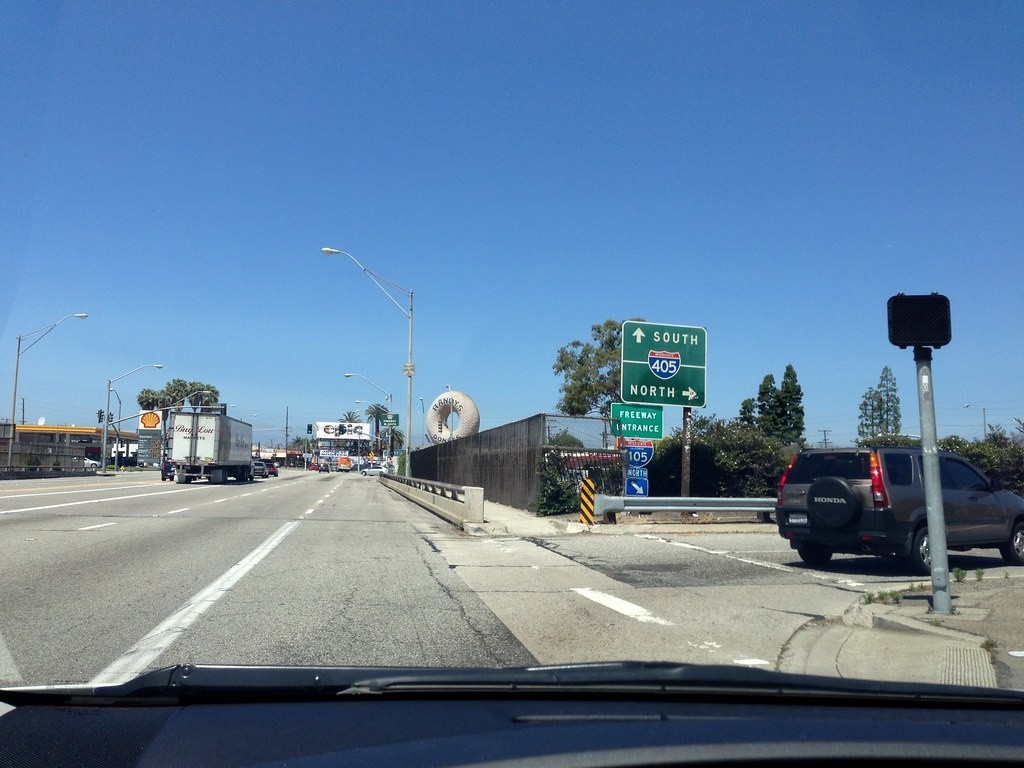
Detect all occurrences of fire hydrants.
[120,465,125,473]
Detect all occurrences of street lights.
[321,247,414,476]
[420,398,425,449]
[445,384,454,441]
[344,374,393,464]
[109,388,122,469]
[102,364,163,473]
[963,404,987,442]
[6,314,89,470]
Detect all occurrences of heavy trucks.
[165,411,256,485]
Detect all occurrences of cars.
[360,464,391,477]
[288,454,368,474]
[265,461,281,477]
[73,456,102,470]
[254,461,270,478]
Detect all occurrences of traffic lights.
[307,423,313,434]
[98,409,104,423]
[109,411,113,422]
[887,290,952,350]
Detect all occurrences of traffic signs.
[621,321,706,408]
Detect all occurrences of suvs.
[775,446,1024,577]
